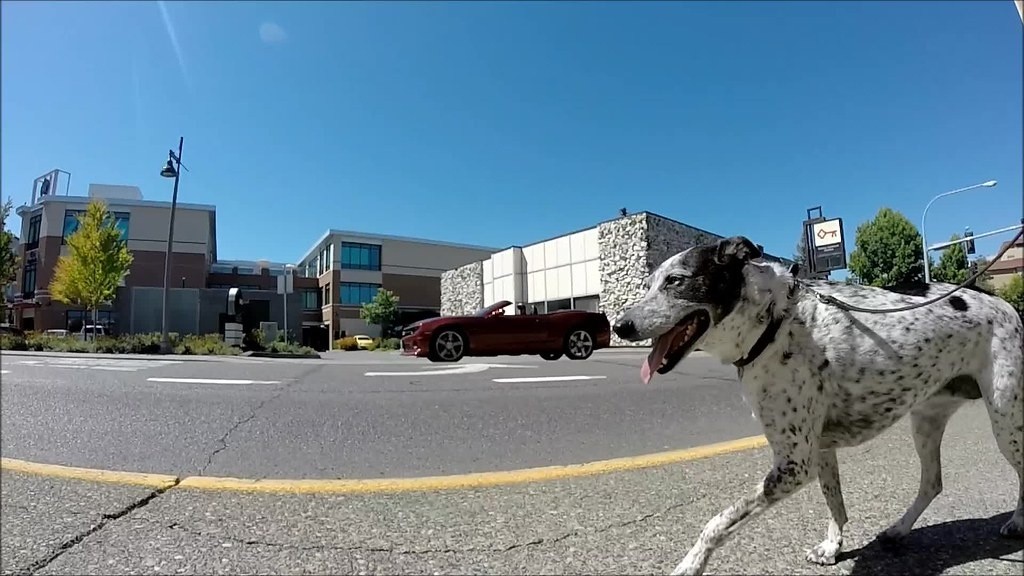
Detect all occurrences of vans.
[81,325,106,340]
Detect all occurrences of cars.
[400,301,613,363]
[44,330,74,340]
[353,335,373,350]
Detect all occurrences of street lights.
[921,180,997,283]
[160,137,186,344]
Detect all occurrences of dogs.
[611,236,1022,575]
